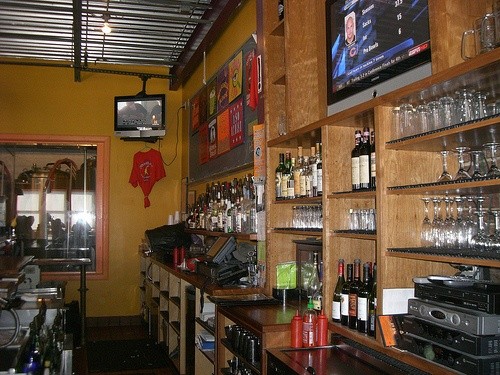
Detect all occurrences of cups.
[290,205,323,229]
[224,323,261,375]
[390,85,500,141]
[348,206,376,233]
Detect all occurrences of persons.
[345,17,356,45]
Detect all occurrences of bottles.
[305,250,324,313]
[184,172,256,234]
[180,244,185,264]
[317,310,329,347]
[331,257,377,334]
[461,1,500,63]
[290,309,303,350]
[173,245,179,263]
[274,141,323,201]
[350,128,377,192]
[21,308,66,375]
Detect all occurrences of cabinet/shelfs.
[139,0,500,375]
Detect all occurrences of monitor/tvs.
[324,0,430,106]
[202,236,234,262]
[113,94,166,137]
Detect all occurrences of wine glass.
[438,139,500,183]
[416,196,500,254]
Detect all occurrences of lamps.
[31,162,71,189]
